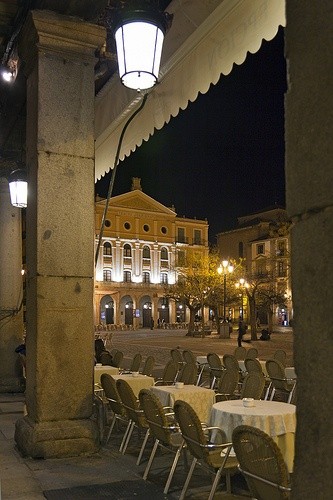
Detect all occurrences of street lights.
[217,260,234,338]
[234,278,250,319]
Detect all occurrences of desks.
[208,400,296,496]
[110,373,155,435]
[196,355,226,385]
[94,365,120,422]
[150,385,216,466]
[284,367,296,404]
[237,360,272,396]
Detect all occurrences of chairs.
[15,320,297,500]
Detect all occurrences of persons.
[237,319,252,348]
[150,317,154,330]
[158,318,165,328]
[194,314,202,322]
[177,318,183,322]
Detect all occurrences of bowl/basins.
[242,397,254,407]
[174,382,184,389]
[132,372,139,377]
[96,363,102,368]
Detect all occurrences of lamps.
[5,111,29,208]
[105,303,109,308]
[107,0,174,92]
[178,304,183,310]
[143,296,147,308]
[161,297,165,310]
[125,296,129,308]
